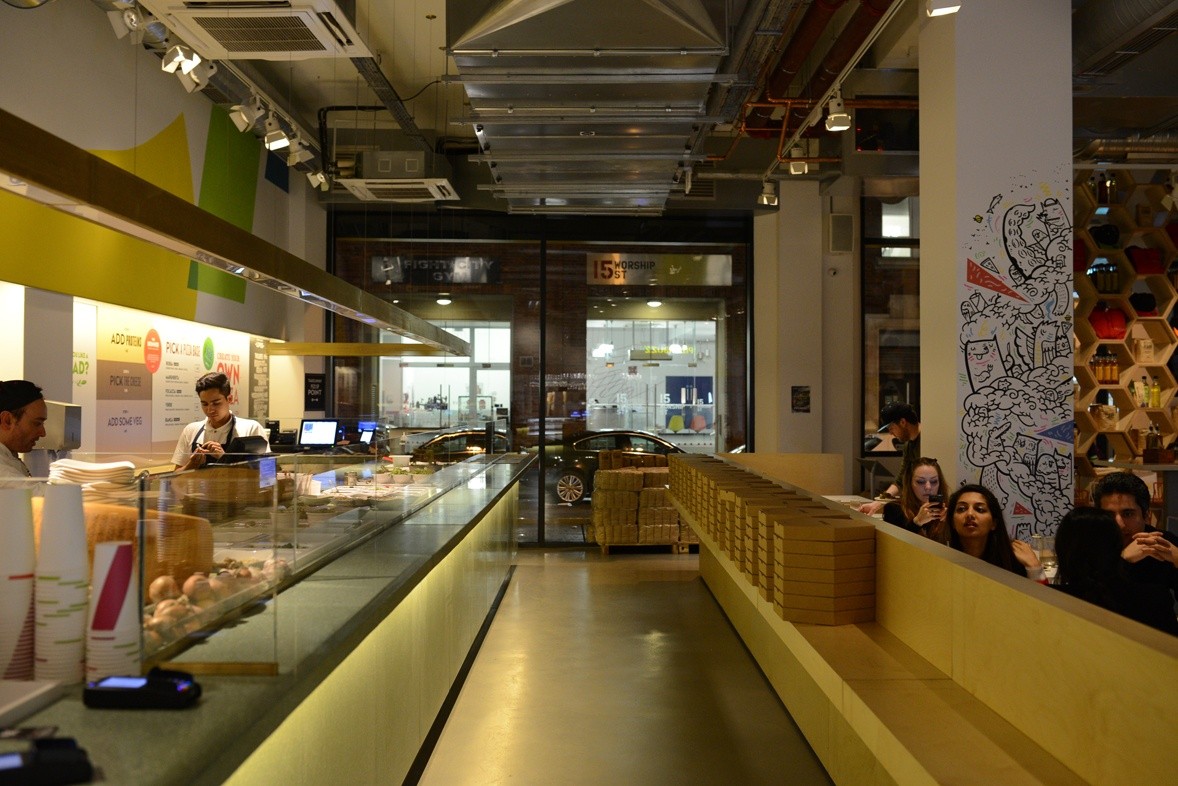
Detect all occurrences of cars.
[866,424,910,454]
[404,428,551,468]
[520,428,690,502]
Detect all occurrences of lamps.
[160,44,202,75]
[305,170,331,192]
[757,182,779,207]
[790,146,807,175]
[175,55,218,94]
[926,0,961,17]
[825,90,851,131]
[286,130,315,167]
[228,90,266,133]
[106,0,152,44]
[263,110,290,151]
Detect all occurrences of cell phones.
[930,494,945,508]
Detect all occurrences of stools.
[858,456,879,501]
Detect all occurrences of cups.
[1,480,141,688]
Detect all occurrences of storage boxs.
[667,452,878,627]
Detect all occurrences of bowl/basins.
[306,510,337,529]
[299,494,405,510]
[388,453,412,468]
[268,511,298,526]
[371,472,432,484]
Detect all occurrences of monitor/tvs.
[298,419,338,448]
[360,431,373,445]
[218,436,268,464]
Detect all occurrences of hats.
[0,379,42,410]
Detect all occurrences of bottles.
[399,432,407,450]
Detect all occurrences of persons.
[859,400,921,516]
[1093,471,1178,636]
[937,484,1050,586]
[882,456,949,545]
[1032,505,1126,615]
[0,380,48,478]
[168,371,273,472]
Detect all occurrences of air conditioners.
[336,151,465,203]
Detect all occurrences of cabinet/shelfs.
[1064,161,1178,480]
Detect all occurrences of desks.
[822,494,892,520]
